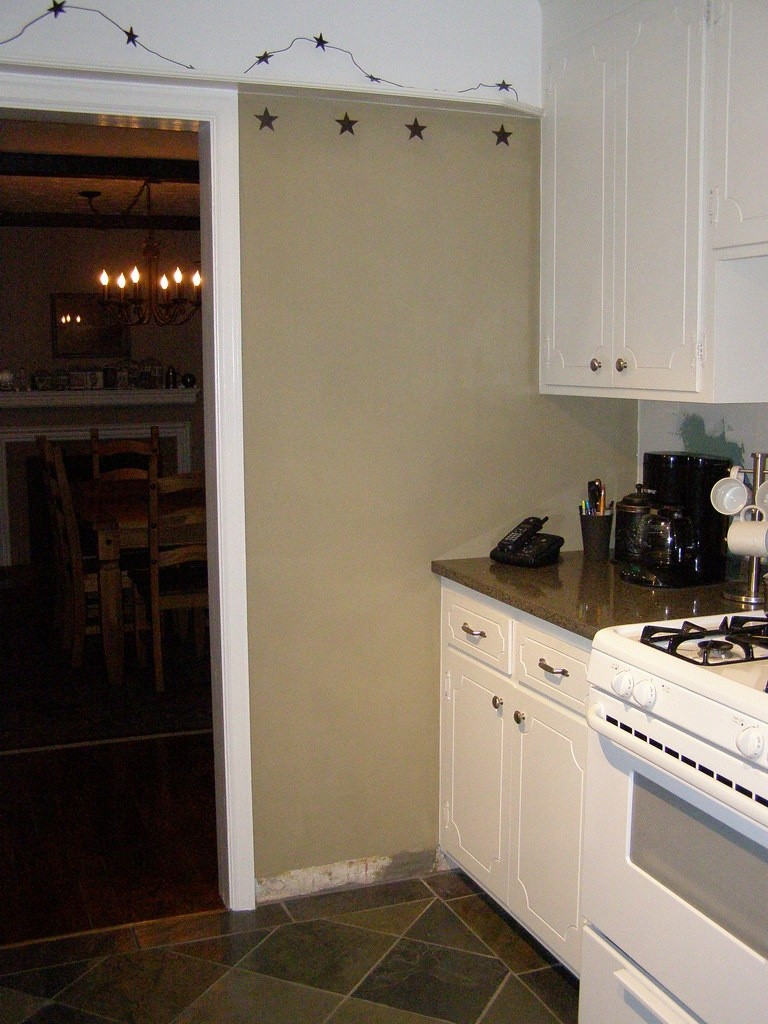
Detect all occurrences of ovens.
[577,687,768,1024]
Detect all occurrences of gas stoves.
[588,610,768,769]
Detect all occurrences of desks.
[0,383,203,568]
[56,471,206,684]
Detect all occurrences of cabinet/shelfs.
[430,572,667,983]
[538,0,768,406]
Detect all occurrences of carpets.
[0,729,230,952]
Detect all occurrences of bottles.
[166,365,176,389]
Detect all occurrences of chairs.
[89,426,160,483]
[34,432,151,671]
[128,455,210,698]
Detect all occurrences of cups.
[728,505,768,556]
[710,465,753,516]
[616,484,653,555]
[580,513,613,561]
[755,481,768,516]
[70,372,86,390]
[86,371,104,389]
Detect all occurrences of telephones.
[490,516,566,568]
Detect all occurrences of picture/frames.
[49,291,133,360]
[65,368,107,391]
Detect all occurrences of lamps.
[77,176,201,327]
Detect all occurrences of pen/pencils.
[600,484,606,516]
[582,500,615,517]
